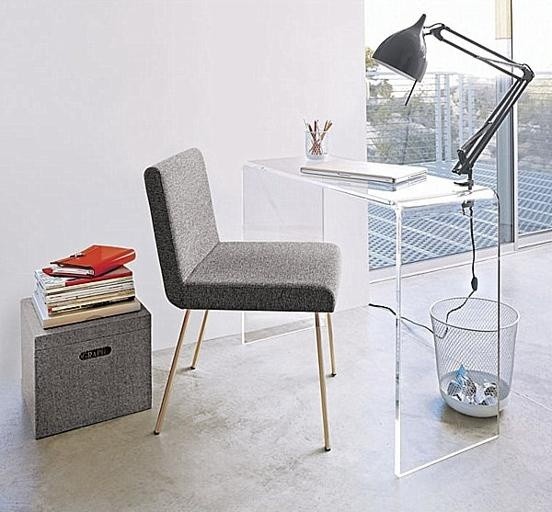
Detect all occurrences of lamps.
[370,12,535,194]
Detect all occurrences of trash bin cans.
[429,297,520,418]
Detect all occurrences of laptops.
[300,159,428,184]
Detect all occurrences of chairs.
[143,148,341,453]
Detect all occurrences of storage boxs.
[20,297,151,440]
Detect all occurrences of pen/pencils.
[307,119,333,156]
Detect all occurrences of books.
[41,244,136,280]
[30,264,142,331]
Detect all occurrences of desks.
[242,155,501,479]
